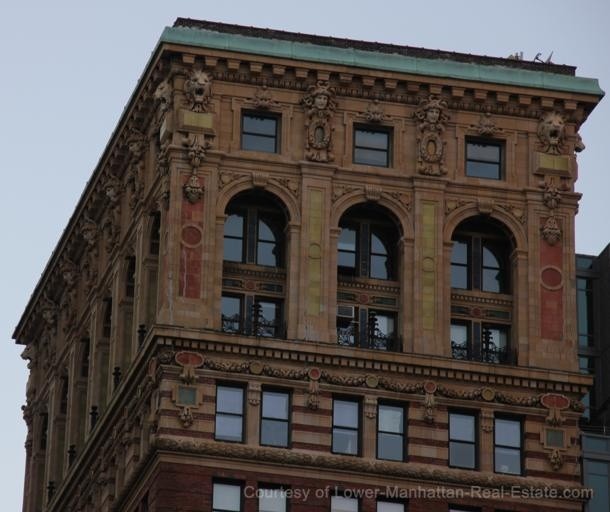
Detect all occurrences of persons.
[38,301,57,324]
[58,267,78,282]
[304,82,336,117]
[79,223,97,245]
[124,135,144,153]
[415,96,447,134]
[102,181,119,200]
